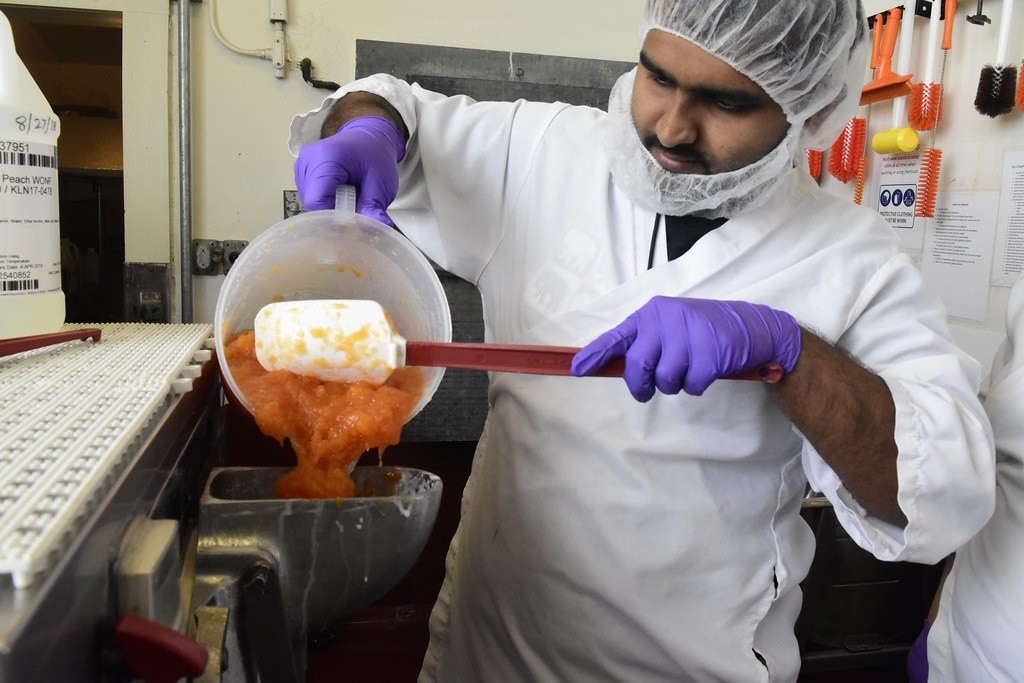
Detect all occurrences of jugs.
[214,184,452,437]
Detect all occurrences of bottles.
[1,9,66,341]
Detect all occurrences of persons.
[285,1,997,683]
[902,234,1024,682]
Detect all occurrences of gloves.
[572,296,802,404]
[294,116,407,227]
